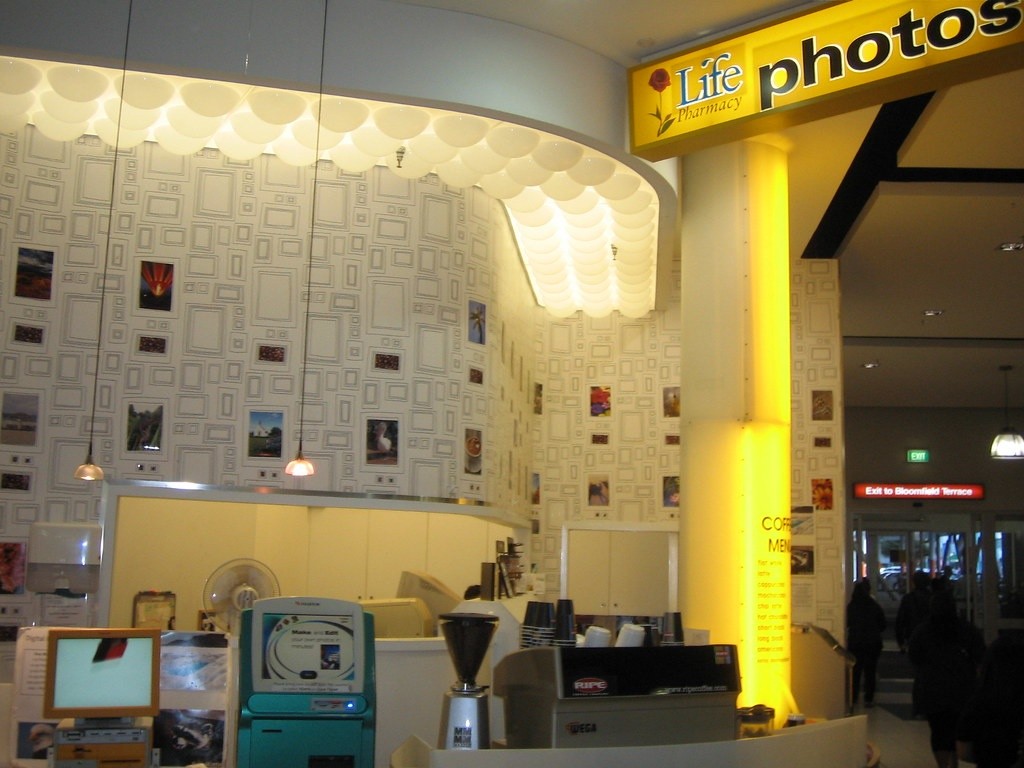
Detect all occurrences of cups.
[555,599,685,646]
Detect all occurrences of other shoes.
[864,700,875,708]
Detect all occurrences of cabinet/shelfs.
[560,519,680,619]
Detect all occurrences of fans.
[199,555,285,634]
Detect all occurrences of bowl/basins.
[521,600,555,647]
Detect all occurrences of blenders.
[437,612,499,751]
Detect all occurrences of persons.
[896,571,957,654]
[847,578,891,713]
[908,615,1024,768]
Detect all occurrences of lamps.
[283,0,333,479]
[68,0,135,483]
[986,363,1024,467]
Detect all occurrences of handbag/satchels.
[865,621,882,656]
